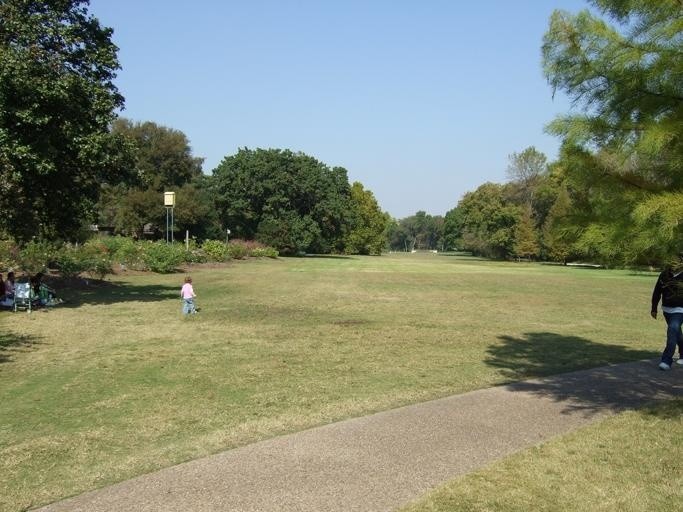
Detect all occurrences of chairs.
[12,275,32,315]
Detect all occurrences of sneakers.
[659,362,671,370]
[676,359,682,365]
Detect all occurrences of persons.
[25,277,41,305]
[5,272,15,299]
[179,276,197,315]
[650,253,683,371]
[34,273,57,302]
[0,273,6,301]
